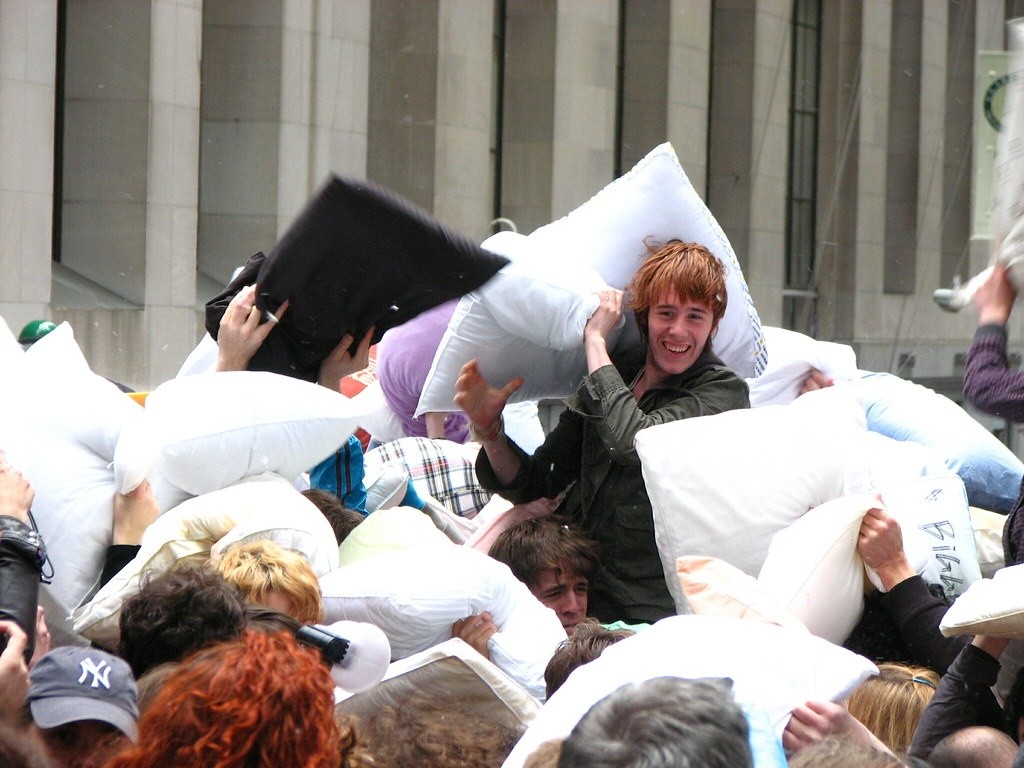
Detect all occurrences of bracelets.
[467,414,505,444]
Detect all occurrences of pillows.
[527,141,769,380]
[848,368,1024,515]
[743,325,858,408]
[317,546,570,704]
[332,637,543,732]
[674,491,930,642]
[205,170,511,383]
[73,471,339,646]
[0,315,116,644]
[24,320,153,495]
[375,298,470,443]
[634,388,984,615]
[411,231,625,421]
[939,563,1024,638]
[144,372,381,517]
[499,614,880,768]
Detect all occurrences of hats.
[25,646,140,745]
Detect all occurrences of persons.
[0,282,1024,768]
[963,259,1024,568]
[454,240,752,624]
